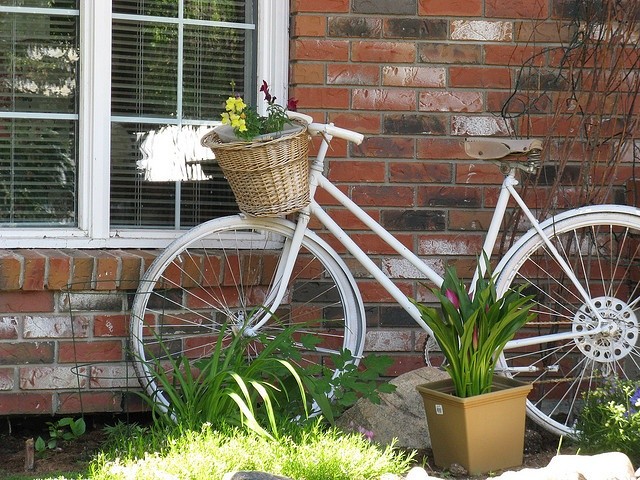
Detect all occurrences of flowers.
[218,77,298,139]
[411,245,540,399]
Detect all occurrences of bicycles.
[127,110,639,448]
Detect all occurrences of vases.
[415,374,534,476]
[213,121,303,142]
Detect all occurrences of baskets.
[201,116,311,217]
